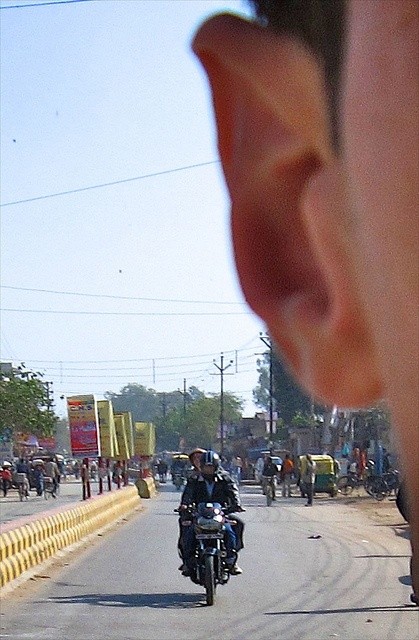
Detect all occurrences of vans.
[298,454,337,499]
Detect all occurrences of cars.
[254,456,284,484]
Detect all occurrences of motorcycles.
[38,474,61,500]
[259,475,282,506]
[15,468,34,502]
[174,502,246,605]
[171,473,186,492]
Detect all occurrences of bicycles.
[364,468,400,500]
[336,461,373,495]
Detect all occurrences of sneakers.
[222,567,229,583]
[181,566,195,576]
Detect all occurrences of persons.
[182,451,241,576]
[155,453,189,482]
[304,455,316,505]
[0,453,98,499]
[280,453,297,498]
[191,0,418,603]
[179,447,245,574]
[233,454,243,485]
[348,439,391,481]
[253,451,279,501]
[330,455,349,497]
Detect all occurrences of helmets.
[200,451,221,482]
[189,447,206,461]
